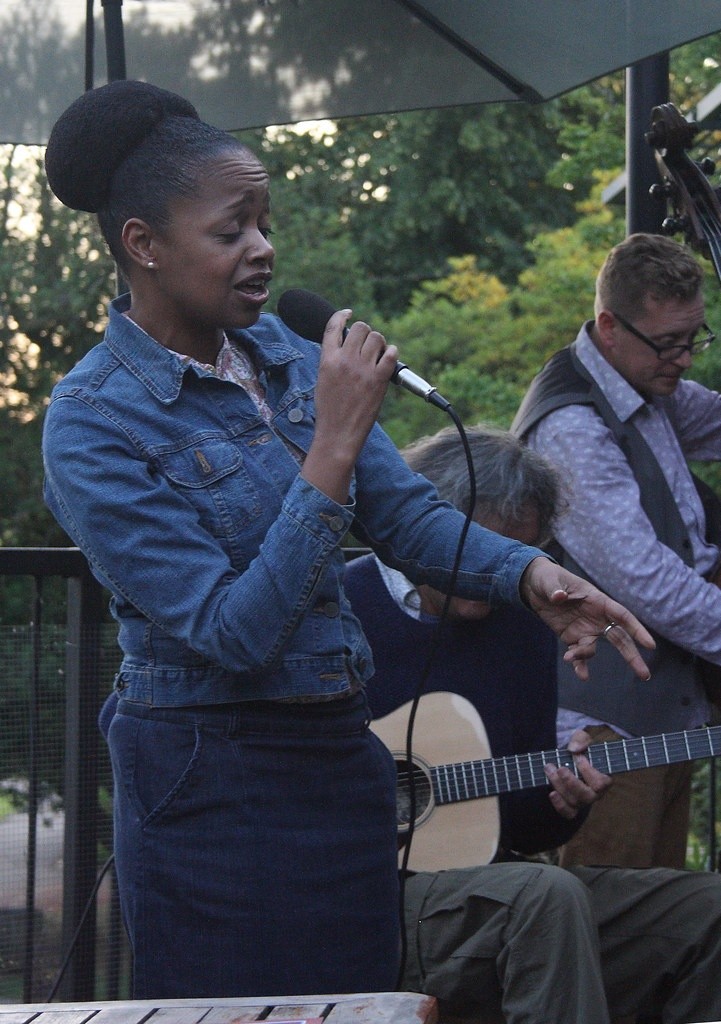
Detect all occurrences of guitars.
[362,690,721,876]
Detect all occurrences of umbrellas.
[0,0,721,147]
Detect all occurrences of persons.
[43,78,657,1002]
[511,232,721,880]
[339,425,721,1023]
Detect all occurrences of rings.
[603,620,614,637]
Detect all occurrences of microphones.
[278,288,451,414]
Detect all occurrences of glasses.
[606,305,717,361]
